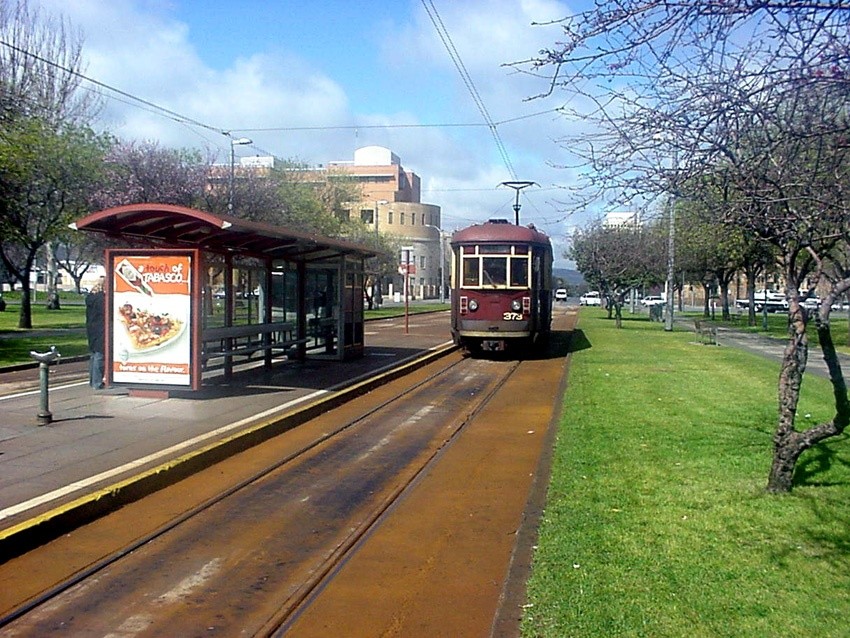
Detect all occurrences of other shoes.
[98,383,114,389]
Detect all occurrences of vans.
[556,288,567,301]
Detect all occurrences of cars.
[215,290,258,300]
[640,296,664,306]
[580,291,601,306]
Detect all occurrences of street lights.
[375,200,390,307]
[424,224,444,303]
[230,138,254,218]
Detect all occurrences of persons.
[86,283,105,388]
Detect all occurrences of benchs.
[694,320,719,346]
[201,317,338,382]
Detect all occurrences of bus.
[449,181,553,358]
